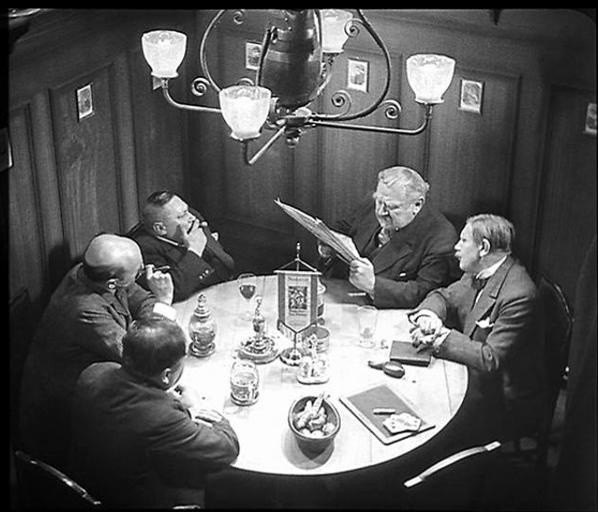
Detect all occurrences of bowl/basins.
[287,393,341,451]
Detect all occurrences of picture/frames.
[580,100,598,137]
[244,40,264,72]
[456,76,484,116]
[74,80,96,123]
[344,57,370,93]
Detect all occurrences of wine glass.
[238,273,259,320]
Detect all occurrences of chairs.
[535,275,572,472]
[12,446,114,511]
[125,219,146,240]
[395,441,510,512]
[9,284,38,409]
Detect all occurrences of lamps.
[137,7,459,168]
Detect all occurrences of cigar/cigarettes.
[143,265,171,273]
[192,221,208,227]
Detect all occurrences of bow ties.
[472,277,486,287]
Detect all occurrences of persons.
[75,316,238,512]
[19,232,175,463]
[408,214,550,431]
[128,191,234,303]
[317,165,464,309]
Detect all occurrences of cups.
[229,358,260,406]
[357,305,378,347]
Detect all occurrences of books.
[274,196,360,266]
[390,340,431,366]
